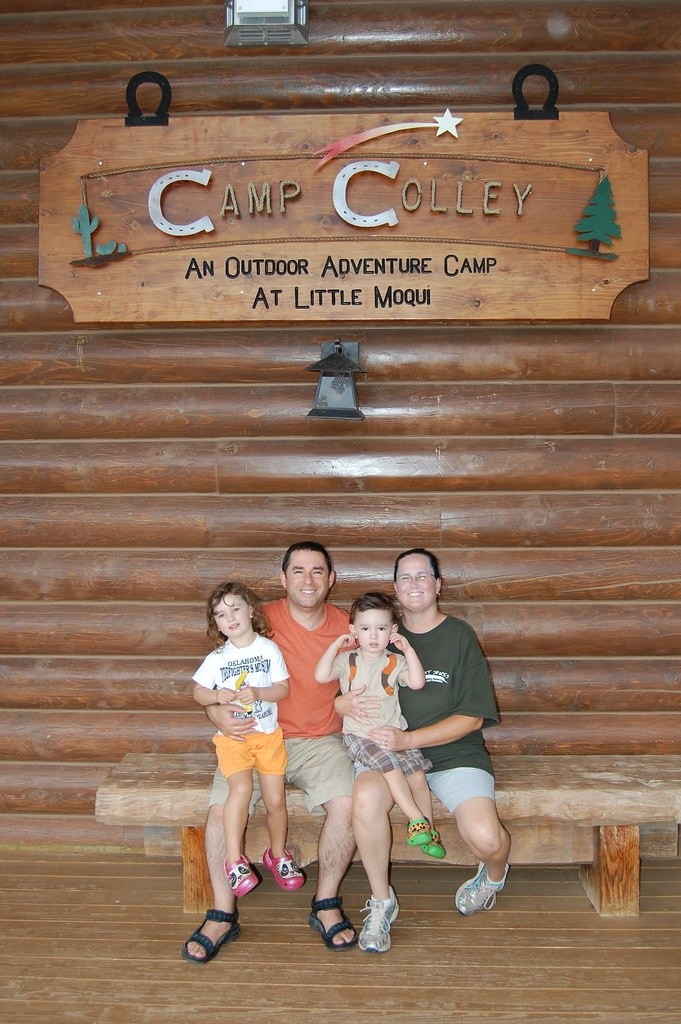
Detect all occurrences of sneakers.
[455,861,509,917]
[358,886,399,952]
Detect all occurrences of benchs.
[95,752,681,917]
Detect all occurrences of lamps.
[224,0,310,48]
[304,339,367,420]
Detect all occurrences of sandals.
[182,909,240,964]
[421,830,444,858]
[406,817,430,846]
[308,894,357,952]
[223,853,258,898]
[263,846,304,891]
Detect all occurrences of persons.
[181,541,356,964]
[316,593,443,858]
[331,549,510,954]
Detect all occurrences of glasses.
[396,574,435,582]
